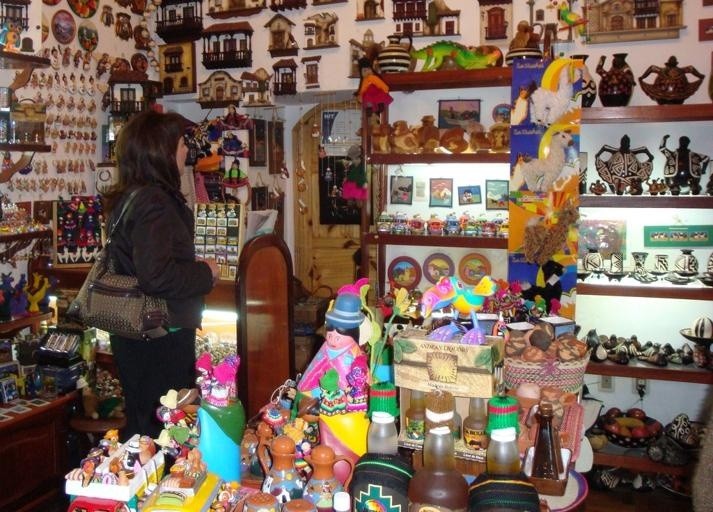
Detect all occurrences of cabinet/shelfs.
[390,328,506,476]
[0,48,56,333]
[361,67,513,323]
[577,104,713,479]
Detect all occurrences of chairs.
[70,350,128,448]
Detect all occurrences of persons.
[105,110,222,473]
[299,292,370,488]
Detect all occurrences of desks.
[0,382,81,512]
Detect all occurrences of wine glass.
[680,327,712,367]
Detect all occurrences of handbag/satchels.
[65,182,175,343]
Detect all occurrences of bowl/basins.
[578,269,712,285]
[597,412,662,448]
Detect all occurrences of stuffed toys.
[522,201,579,265]
[516,127,573,192]
[525,64,582,125]
[369,110,510,156]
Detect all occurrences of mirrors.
[319,109,372,225]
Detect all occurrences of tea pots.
[256,427,354,511]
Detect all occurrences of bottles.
[405,389,489,512]
[485,394,520,476]
[531,403,563,480]
[366,382,397,456]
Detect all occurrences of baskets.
[499,349,592,397]
